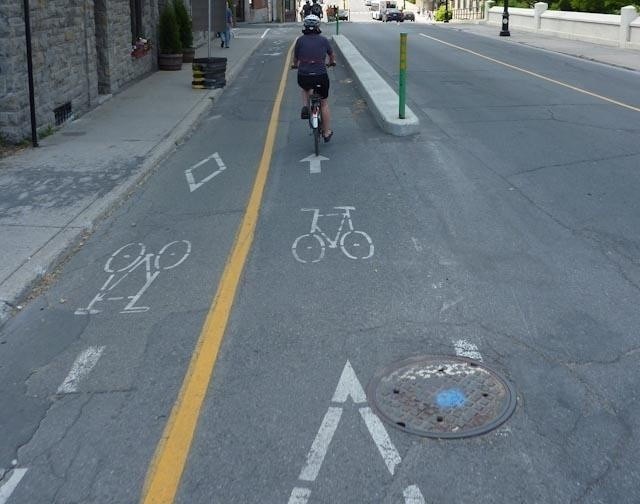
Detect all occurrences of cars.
[402,11,415,22]
[337,9,348,21]
[364,0,373,6]
[381,9,404,23]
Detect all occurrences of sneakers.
[324,128,333,142]
[301,106,308,119]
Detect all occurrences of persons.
[218,1,233,48]
[292,14,335,143]
[326,4,337,22]
[303,0,324,22]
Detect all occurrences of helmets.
[304,14,321,27]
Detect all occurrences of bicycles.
[299,11,323,35]
[290,60,335,155]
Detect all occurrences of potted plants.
[171,0,197,64]
[157,2,185,70]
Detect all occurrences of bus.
[368,0,398,20]
